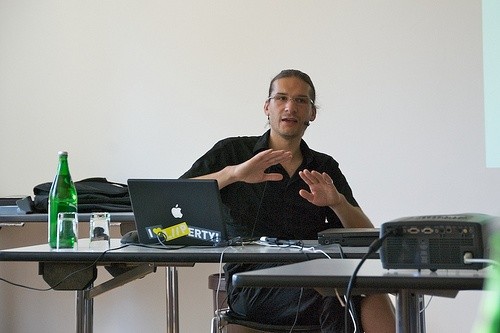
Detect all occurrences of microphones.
[304,121,310,126]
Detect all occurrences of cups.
[56,212,77,249]
[89,212,111,250]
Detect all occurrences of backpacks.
[33,176,133,213]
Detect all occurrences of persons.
[175,69,397,333]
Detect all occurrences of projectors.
[380,213,500,269]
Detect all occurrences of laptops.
[126,178,250,246]
[317,228,381,247]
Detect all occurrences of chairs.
[211,307,319,333]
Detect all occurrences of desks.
[0,205,490,333]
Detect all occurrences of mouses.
[121,230,138,244]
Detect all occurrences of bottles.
[47,151,78,248]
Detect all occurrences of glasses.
[268,95,315,104]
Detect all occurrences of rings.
[326,180,333,185]
